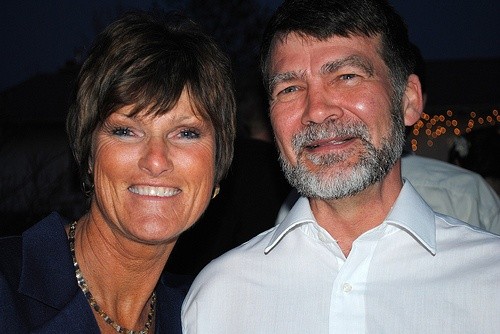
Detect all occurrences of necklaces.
[70,220,155,334]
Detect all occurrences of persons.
[181,0,500,334]
[0,7,237,334]
